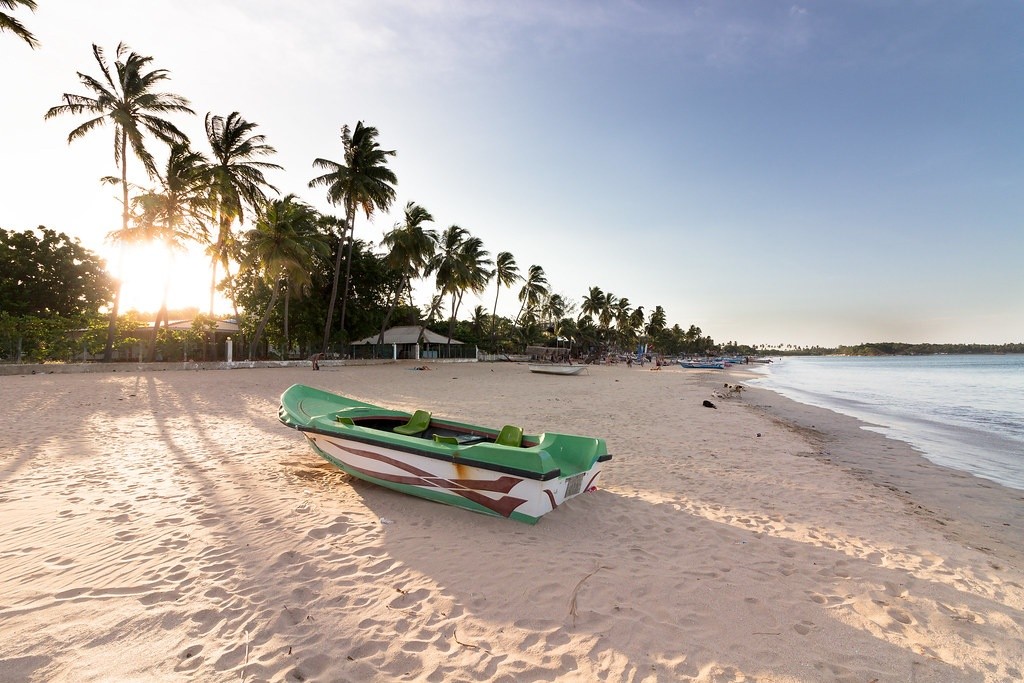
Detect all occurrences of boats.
[274,383,613,525]
[528,363,593,376]
[677,357,740,369]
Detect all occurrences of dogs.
[724,382,727,388]
[728,384,748,398]
[703,401,717,410]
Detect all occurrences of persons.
[414,365,431,371]
[311,353,325,370]
[537,352,755,371]
